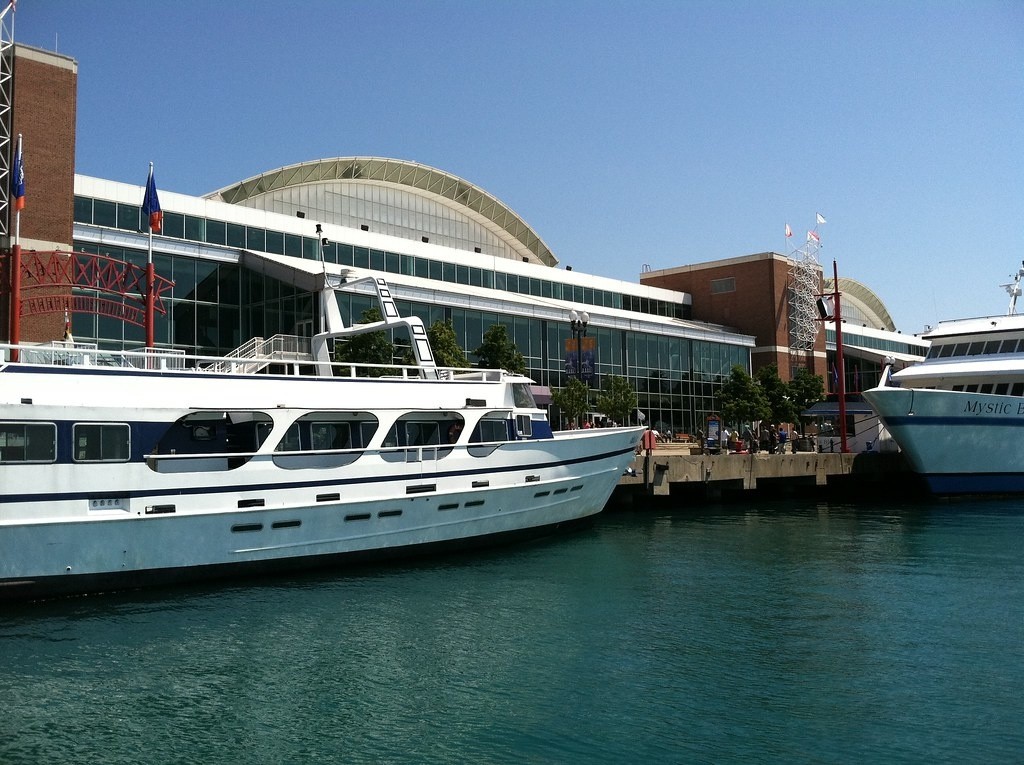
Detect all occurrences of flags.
[809,230,820,241]
[141,172,163,232]
[785,224,792,237]
[12,144,25,211]
[832,366,838,384]
[817,213,827,224]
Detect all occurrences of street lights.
[569,308,589,429]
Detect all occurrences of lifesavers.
[448,424,464,442]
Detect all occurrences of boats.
[0,224,652,601]
[860,260,1024,501]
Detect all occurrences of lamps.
[475,247,481,253]
[361,225,368,231]
[422,236,428,242]
[566,266,572,270]
[297,211,305,218]
[523,257,528,262]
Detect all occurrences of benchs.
[676,434,690,439]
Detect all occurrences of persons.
[697,426,703,448]
[721,425,799,455]
[566,418,618,430]
[651,426,671,443]
